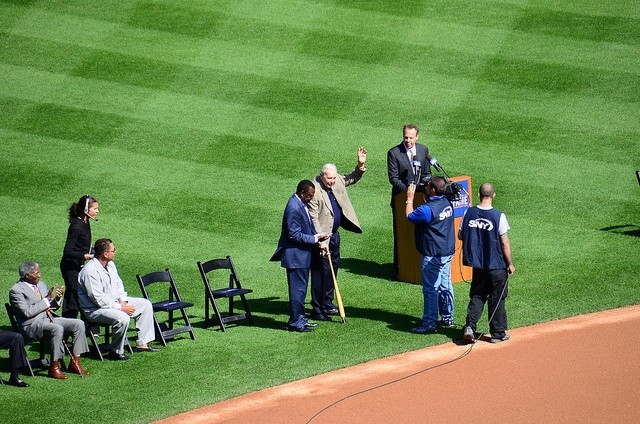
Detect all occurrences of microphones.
[85,212,99,222]
[426,155,440,172]
[412,155,420,176]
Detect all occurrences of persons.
[0,330,30,387]
[78,238,161,360]
[458,182,515,342]
[60,195,100,347]
[307,147,367,321]
[9,261,91,379]
[406,176,456,333]
[269,180,331,332]
[387,125,432,280]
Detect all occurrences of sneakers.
[463,326,476,344]
[411,324,435,334]
[490,334,509,343]
[437,319,452,328]
[93,333,99,337]
[65,341,71,347]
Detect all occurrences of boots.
[48,360,68,378]
[68,355,89,374]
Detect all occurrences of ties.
[33,285,42,298]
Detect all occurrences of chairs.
[68,283,134,362]
[196,254,254,333]
[135,267,197,347]
[5,302,68,378]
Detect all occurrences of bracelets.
[405,201,413,204]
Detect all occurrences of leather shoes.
[109,349,129,360]
[323,302,338,310]
[311,311,332,321]
[134,345,162,352]
[306,322,318,328]
[294,327,311,332]
[9,378,29,387]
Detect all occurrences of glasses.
[104,248,116,254]
